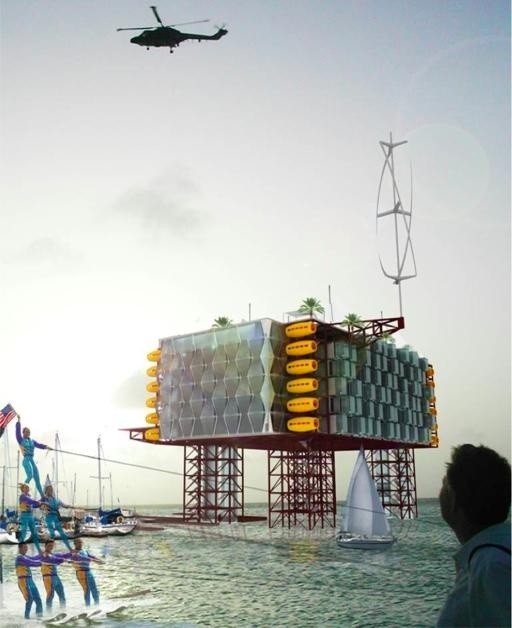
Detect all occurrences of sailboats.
[338,449,397,552]
[1,432,139,543]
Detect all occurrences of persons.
[18,483,49,558]
[434,444,511,628]
[40,538,75,615]
[15,413,55,501]
[38,484,77,555]
[15,541,51,621]
[70,536,106,606]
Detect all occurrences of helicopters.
[118,5,228,53]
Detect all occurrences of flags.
[0,403,18,432]
